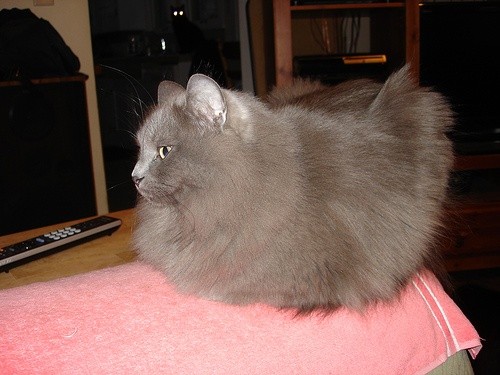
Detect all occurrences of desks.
[0,208,136,290]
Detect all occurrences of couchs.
[0,260,482,375]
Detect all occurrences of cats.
[131,61,458,311]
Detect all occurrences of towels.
[1,248,488,372]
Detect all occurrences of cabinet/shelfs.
[273,0,420,89]
[427,154,500,271]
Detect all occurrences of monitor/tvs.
[419,2,500,153]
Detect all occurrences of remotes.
[0,215,122,273]
